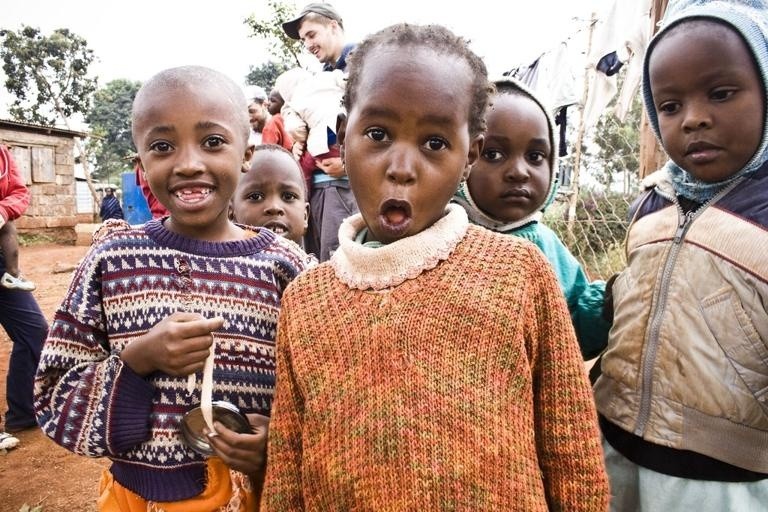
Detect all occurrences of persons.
[0,141,50,454]
[589,0,768,512]
[258,21,613,512]
[452,76,608,362]
[34,0,364,511]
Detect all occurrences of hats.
[282,2,343,40]
[243,85,268,107]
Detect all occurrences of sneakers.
[0,431,21,452]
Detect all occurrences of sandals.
[0,271,37,291]
